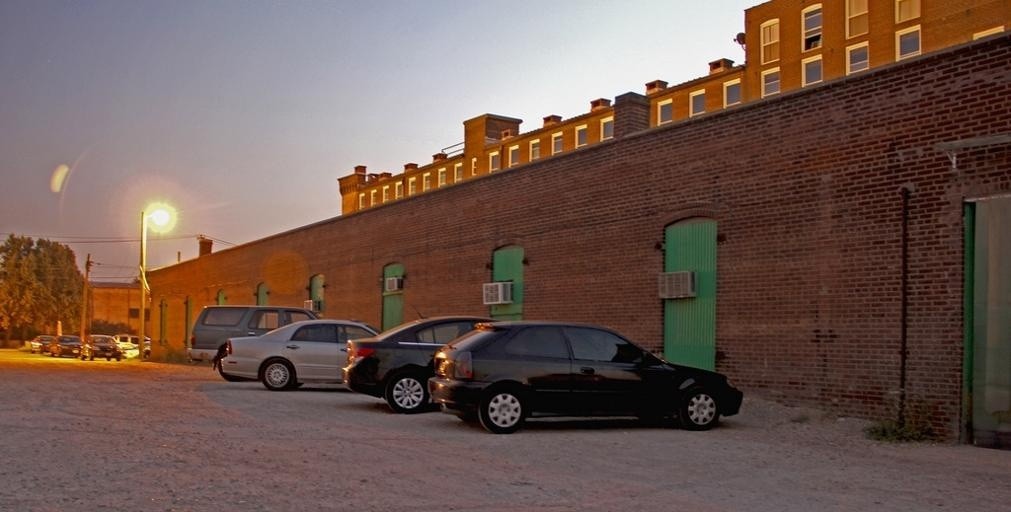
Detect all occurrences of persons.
[81,340,92,361]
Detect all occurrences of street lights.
[138,209,175,360]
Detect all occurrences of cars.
[340,315,502,415]
[222,318,382,391]
[426,319,743,433]
[30,331,151,362]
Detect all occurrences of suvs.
[189,303,321,382]
